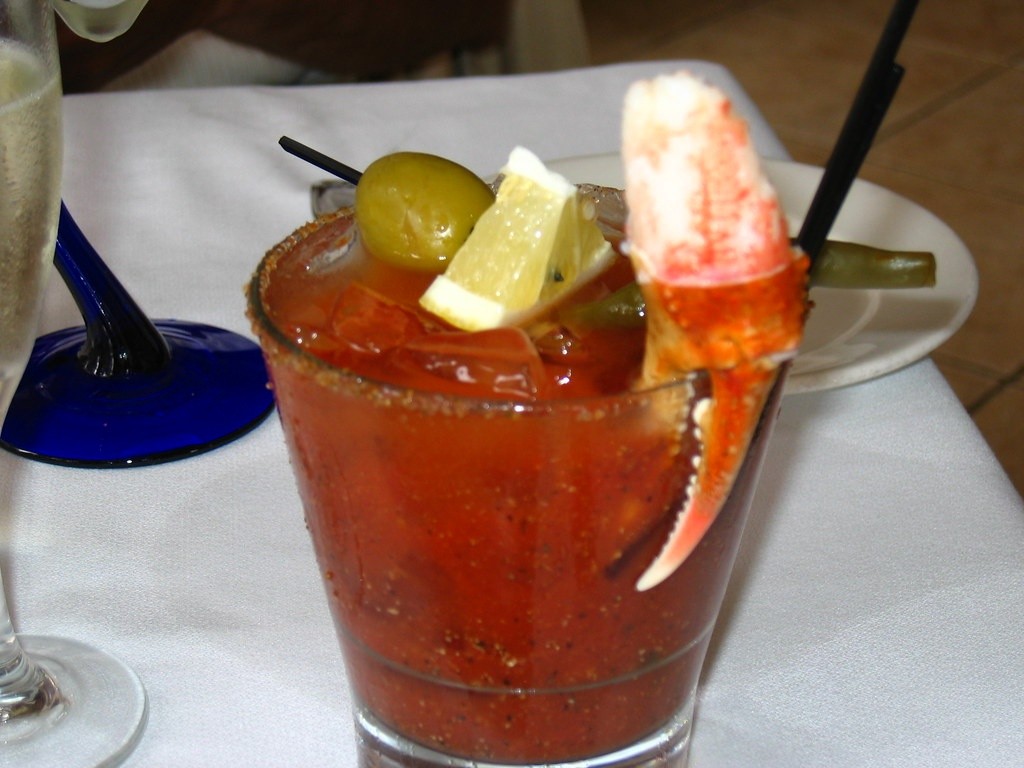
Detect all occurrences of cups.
[249,180,810,768]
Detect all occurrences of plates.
[486,153,980,393]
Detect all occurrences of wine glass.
[0,0,146,768]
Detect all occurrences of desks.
[0,59,1024,768]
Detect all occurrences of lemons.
[420,145,618,332]
